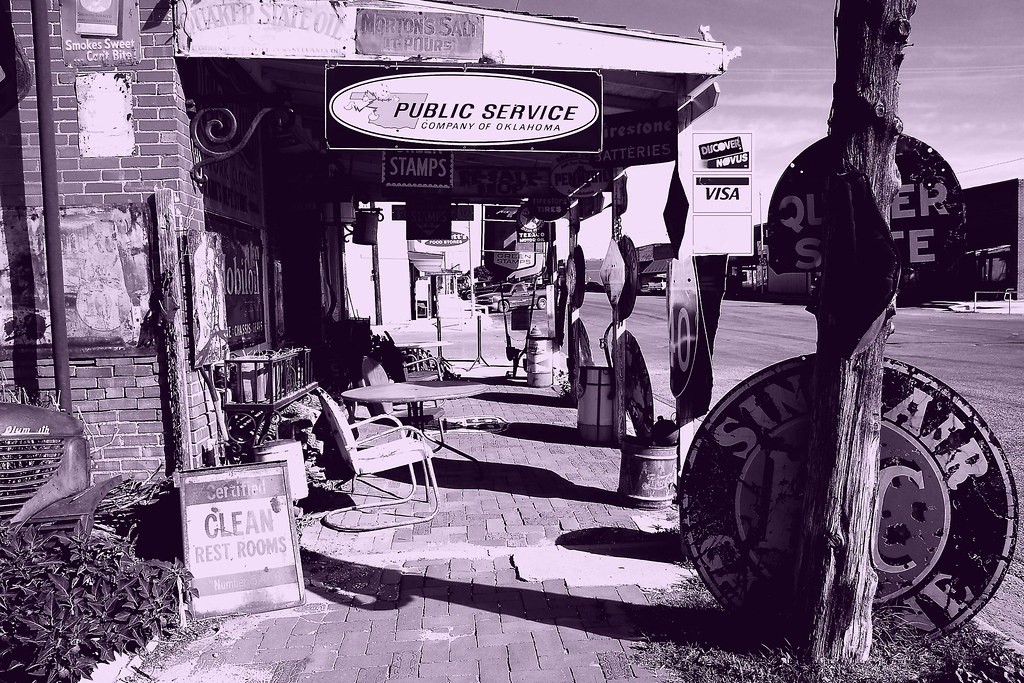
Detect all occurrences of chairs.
[318,387,442,532]
[360,358,445,455]
[379,340,445,383]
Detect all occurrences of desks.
[339,381,491,469]
[394,342,451,372]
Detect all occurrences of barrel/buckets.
[617,435,679,509]
[525,334,553,387]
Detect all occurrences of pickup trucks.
[477,282,547,313]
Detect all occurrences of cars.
[584,281,605,293]
[459,280,498,301]
[525,283,546,289]
